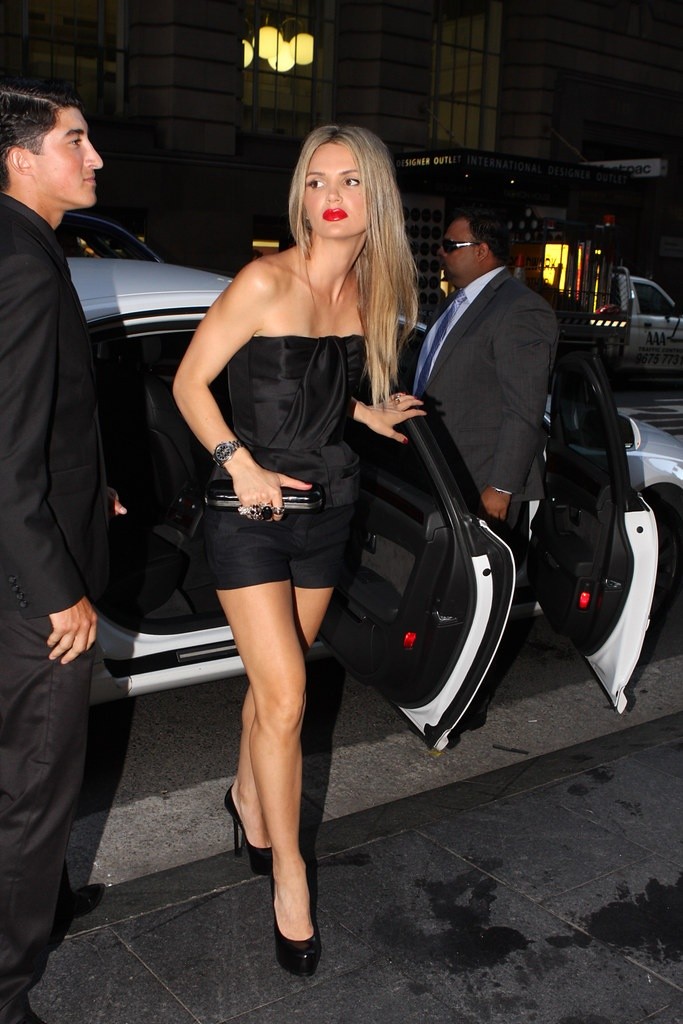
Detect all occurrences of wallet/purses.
[204,479,324,515]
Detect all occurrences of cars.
[55,212,683,752]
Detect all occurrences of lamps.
[241,10,315,73]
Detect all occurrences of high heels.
[270,864,321,977]
[224,783,274,876]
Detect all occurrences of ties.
[415,290,467,397]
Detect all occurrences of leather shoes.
[55,883,102,918]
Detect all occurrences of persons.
[406,207,558,720]
[174,124,426,978]
[0,82,127,1024]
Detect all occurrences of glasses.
[441,239,479,253]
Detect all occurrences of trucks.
[541,266,683,387]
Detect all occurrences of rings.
[238,505,285,521]
[394,397,400,402]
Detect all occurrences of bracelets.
[495,490,505,493]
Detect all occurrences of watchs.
[213,439,243,467]
